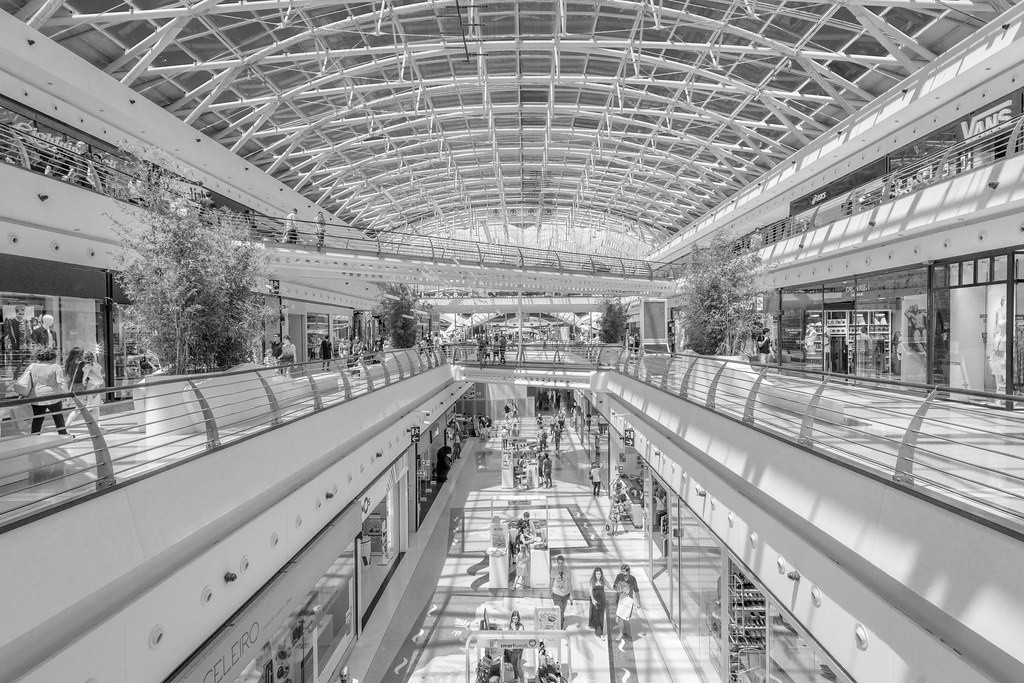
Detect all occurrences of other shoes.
[601,634,606,641]
[616,634,623,642]
[100,427,107,435]
[624,631,632,638]
[88,407,93,412]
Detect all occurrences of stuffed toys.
[436,446,452,483]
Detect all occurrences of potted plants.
[675,226,779,395]
[595,292,632,366]
[100,137,290,437]
[374,280,439,371]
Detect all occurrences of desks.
[272,614,334,683]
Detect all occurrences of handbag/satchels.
[13,363,35,397]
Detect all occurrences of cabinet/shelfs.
[825,309,847,362]
[731,588,766,654]
[847,309,869,367]
[868,308,892,374]
[113,341,140,380]
[804,310,825,369]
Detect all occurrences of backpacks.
[66,397,76,406]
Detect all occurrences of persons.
[31,315,57,347]
[0,131,108,195]
[667,332,675,353]
[3,305,33,367]
[18,346,104,438]
[314,212,327,246]
[857,326,872,377]
[892,303,927,358]
[996,295,1006,325]
[128,172,250,241]
[589,460,667,536]
[264,333,394,379]
[757,328,776,379]
[858,171,931,206]
[449,392,580,488]
[283,208,298,244]
[415,331,513,365]
[335,665,359,683]
[991,320,1006,394]
[139,352,156,381]
[628,333,641,354]
[513,329,601,343]
[807,326,831,355]
[24,317,42,346]
[731,228,763,257]
[508,511,640,683]
